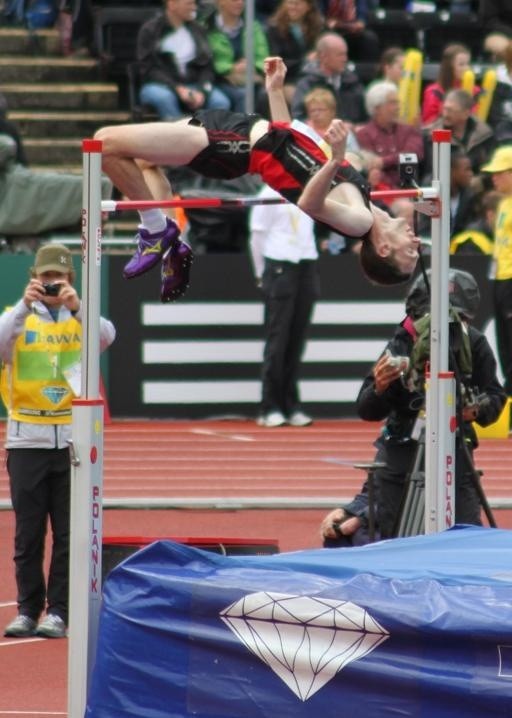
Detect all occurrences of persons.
[478,146,512,417]
[93,55,423,305]
[1,1,511,257]
[0,241,121,639]
[451,187,501,364]
[319,266,510,548]
[248,180,323,428]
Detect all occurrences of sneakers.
[158,240,193,304]
[255,411,287,428]
[122,216,181,281]
[283,410,313,426]
[35,614,68,638]
[3,614,38,637]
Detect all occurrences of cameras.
[40,283,63,296]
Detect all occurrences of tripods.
[392,419,498,542]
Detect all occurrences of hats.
[480,145,512,175]
[34,244,73,276]
